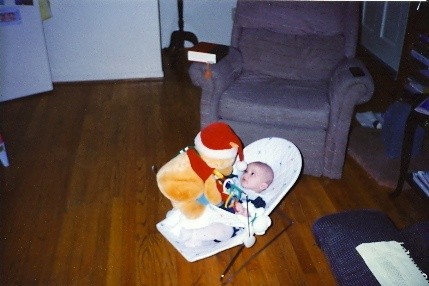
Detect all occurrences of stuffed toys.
[156,123,248,220]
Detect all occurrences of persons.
[169,161,275,243]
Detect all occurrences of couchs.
[188,0,375,181]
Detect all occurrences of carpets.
[347,75,429,191]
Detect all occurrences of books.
[188,42,229,64]
[412,170,429,199]
[415,98,429,115]
